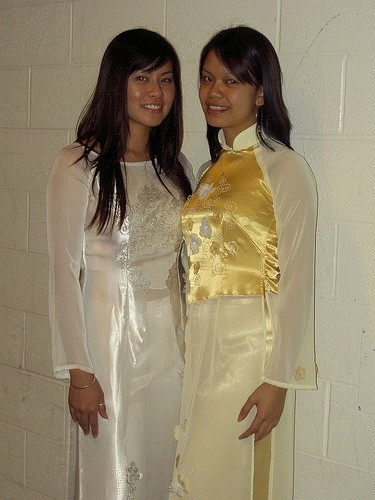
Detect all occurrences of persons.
[175,24,319,500]
[43,28,202,500]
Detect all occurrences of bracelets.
[69,374,97,389]
